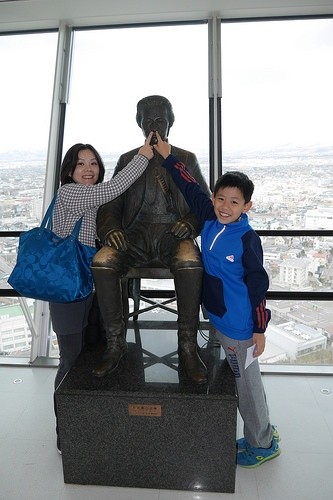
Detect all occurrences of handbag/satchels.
[7,193,95,303]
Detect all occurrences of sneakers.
[235,437,281,469]
[236,429,280,449]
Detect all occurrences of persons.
[49,131,142,455]
[88,96,205,377]
[153,130,280,469]
